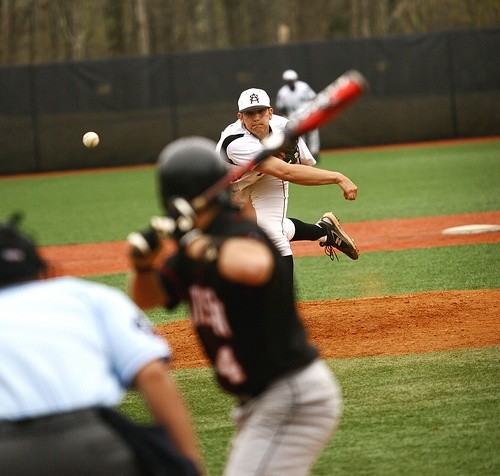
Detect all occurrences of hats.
[0,227,46,285]
[282,70,299,81]
[238,87,271,113]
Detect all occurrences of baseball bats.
[122,67,371,259]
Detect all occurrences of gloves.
[125,228,163,272]
[151,198,202,249]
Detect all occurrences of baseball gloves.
[270,136,302,164]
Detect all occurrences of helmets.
[155,136,231,219]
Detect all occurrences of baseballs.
[81,130,100,149]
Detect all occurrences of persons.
[275,70,320,162]
[0,208,211,475]
[126,137,342,476]
[213,87,359,271]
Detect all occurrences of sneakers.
[316,212,359,262]
